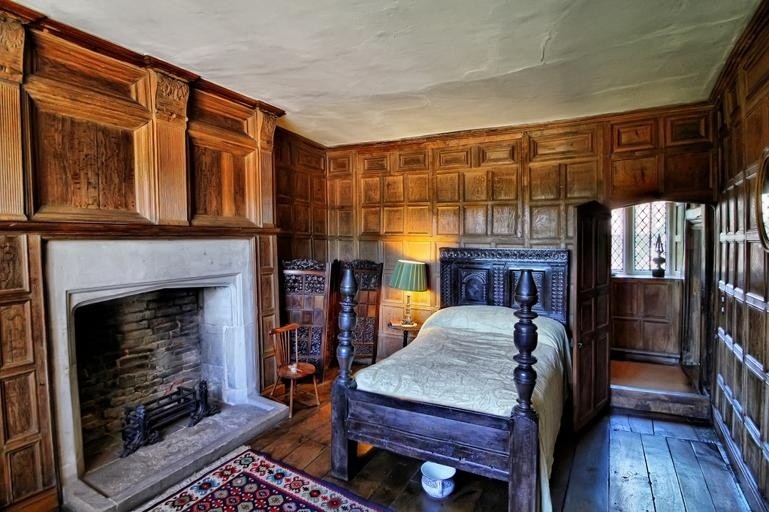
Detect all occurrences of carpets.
[126,445,394,512]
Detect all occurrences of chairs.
[270,323,321,418]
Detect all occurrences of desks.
[387,321,422,347]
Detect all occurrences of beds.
[331,249,569,512]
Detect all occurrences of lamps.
[388,258,427,328]
